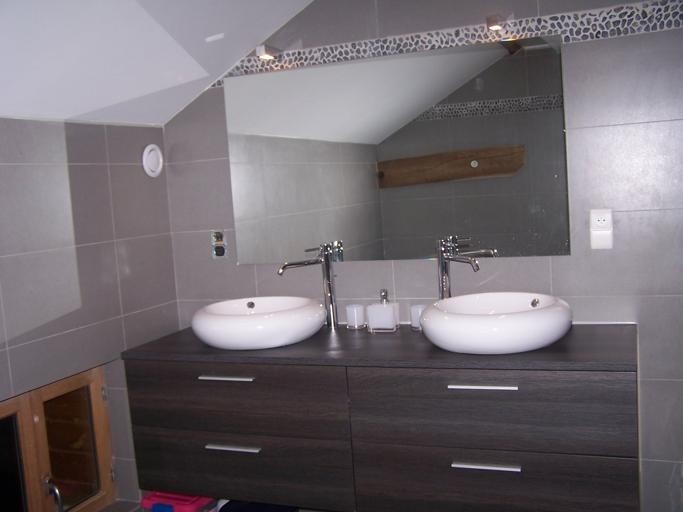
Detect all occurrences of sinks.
[191,296,328,352]
[419,291,573,356]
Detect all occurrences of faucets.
[435,239,481,300]
[277,242,340,328]
[444,233,500,257]
[304,239,345,262]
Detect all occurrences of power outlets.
[589,209,613,232]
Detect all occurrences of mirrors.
[221,35,571,268]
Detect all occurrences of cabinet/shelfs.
[126,363,636,512]
[0,366,116,511]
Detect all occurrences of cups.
[411,305,429,331]
[345,305,366,330]
